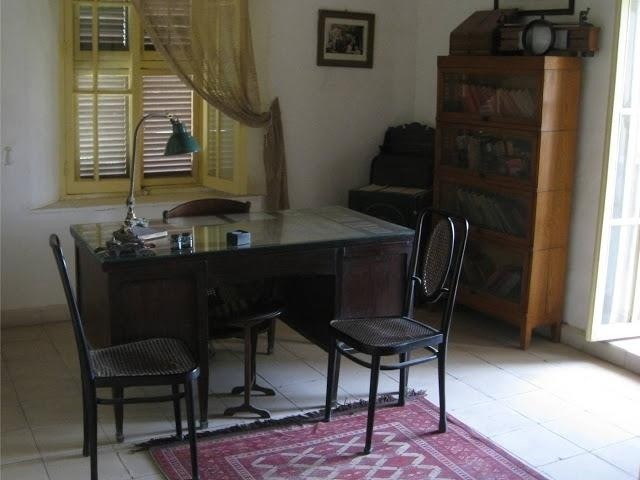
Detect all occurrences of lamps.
[111,113,202,243]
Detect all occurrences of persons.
[330,25,355,51]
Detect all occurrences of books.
[455,128,531,175]
[455,184,532,236]
[133,225,169,240]
[457,82,538,120]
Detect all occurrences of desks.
[69,203,416,445]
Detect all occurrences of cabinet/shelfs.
[428,54,584,349]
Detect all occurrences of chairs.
[49,234,202,480]
[161,198,276,355]
[324,211,470,454]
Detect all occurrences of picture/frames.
[316,7,377,70]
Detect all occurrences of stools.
[208,299,284,419]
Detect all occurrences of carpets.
[135,388,544,480]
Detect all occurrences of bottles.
[181,232,193,248]
[170,234,182,250]
